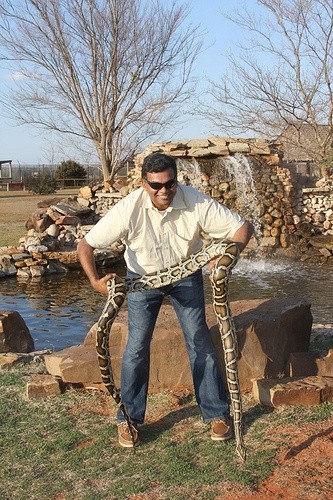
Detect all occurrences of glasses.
[146,177,176,190]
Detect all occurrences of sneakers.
[211,417,232,441]
[117,421,140,447]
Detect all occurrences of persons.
[77,153,254,448]
